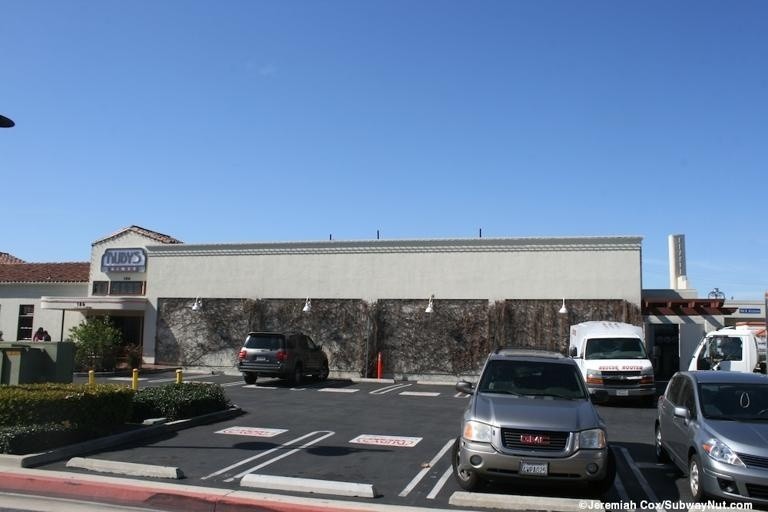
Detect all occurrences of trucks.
[568,321,657,403]
[684,292,768,377]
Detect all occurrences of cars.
[652,369,768,504]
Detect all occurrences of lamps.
[559,298,568,313]
[193,295,202,310]
[302,296,311,311]
[425,295,435,312]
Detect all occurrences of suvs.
[235,330,331,384]
[453,345,616,493]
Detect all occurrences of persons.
[42,330,51,341]
[33,327,43,341]
